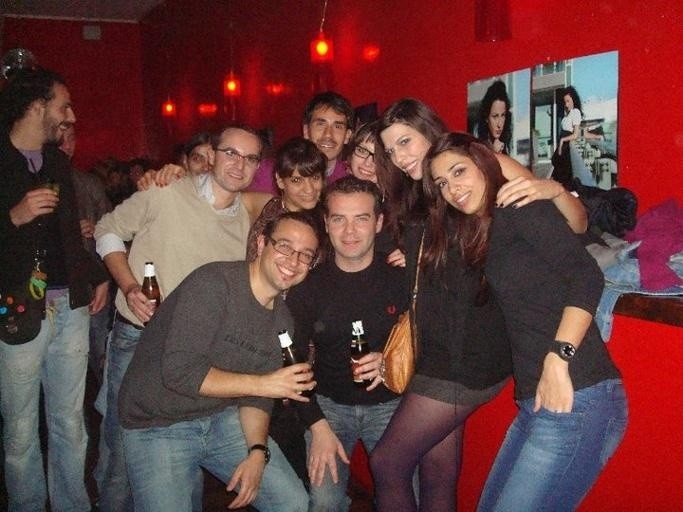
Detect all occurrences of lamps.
[224,22,242,96]
[310,1,336,65]
[474,0,512,43]
[162,94,177,117]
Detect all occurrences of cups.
[34,179,62,216]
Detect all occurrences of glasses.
[352,142,378,162]
[213,144,260,166]
[265,235,317,265]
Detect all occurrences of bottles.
[141,260,164,327]
[349,316,373,390]
[5,49,34,86]
[275,328,317,400]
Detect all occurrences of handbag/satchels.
[1,278,46,347]
[381,226,428,396]
